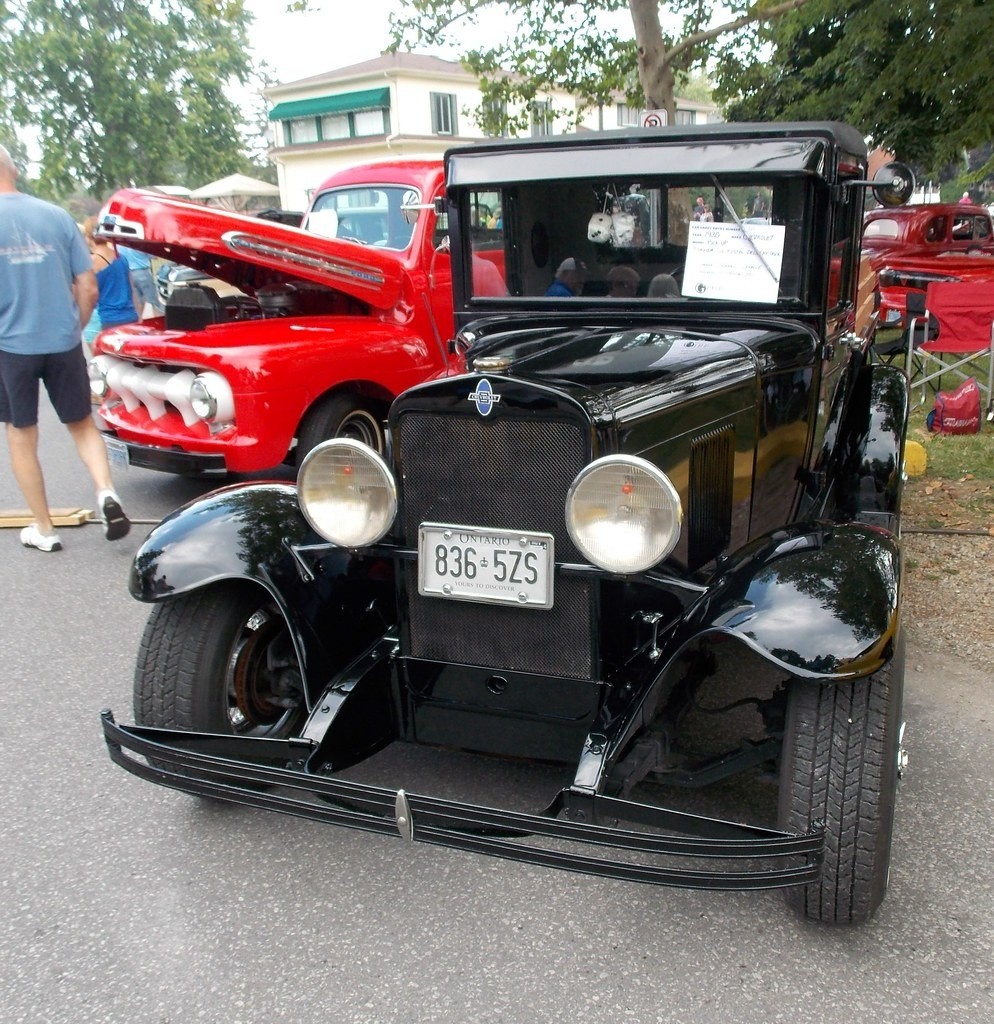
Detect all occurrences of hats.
[555,256,588,278]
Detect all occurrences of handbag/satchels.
[928,376,981,434]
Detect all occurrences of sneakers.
[98,490,131,541]
[20,523,63,552]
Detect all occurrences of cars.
[432,201,504,253]
[154,208,391,314]
[827,204,994,329]
[85,162,506,475]
[99,116,911,925]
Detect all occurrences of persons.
[959,192,971,204]
[542,256,589,298]
[647,274,681,299]
[752,190,765,218]
[0,141,132,553]
[606,266,640,298]
[70,217,165,353]
[690,195,716,222]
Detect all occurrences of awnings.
[267,87,395,122]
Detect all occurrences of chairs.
[866,291,941,396]
[906,282,994,421]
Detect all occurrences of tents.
[149,171,281,197]
